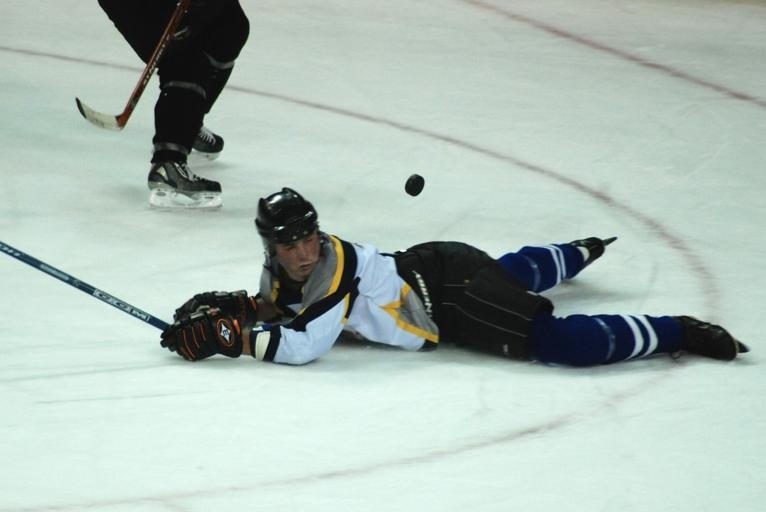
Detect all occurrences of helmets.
[254,187,317,243]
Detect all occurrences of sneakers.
[569,235,605,262]
[148,125,224,197]
[670,314,748,361]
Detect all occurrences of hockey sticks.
[76,0,188,132]
[0,242,273,333]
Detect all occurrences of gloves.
[160,289,258,362]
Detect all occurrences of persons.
[161,188,750,365]
[98,0,250,211]
